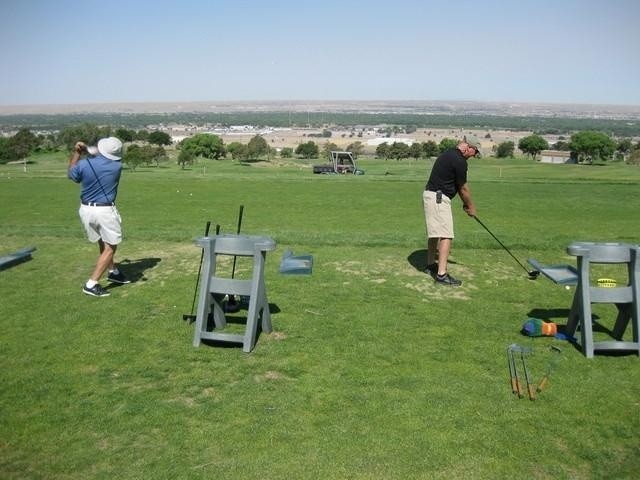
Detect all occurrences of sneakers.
[106,268,131,284]
[82,282,111,297]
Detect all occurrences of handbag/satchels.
[436,191,442,203]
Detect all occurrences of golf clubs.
[183,205,244,320]
[508,344,561,401]
[81,148,121,224]
[463,205,540,276]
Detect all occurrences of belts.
[81,202,115,206]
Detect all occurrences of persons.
[421,133,477,285]
[67,136,131,297]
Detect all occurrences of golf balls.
[565,285,570,290]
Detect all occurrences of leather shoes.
[425,263,438,273]
[436,274,461,285]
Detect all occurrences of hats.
[98,137,123,161]
[463,134,484,159]
